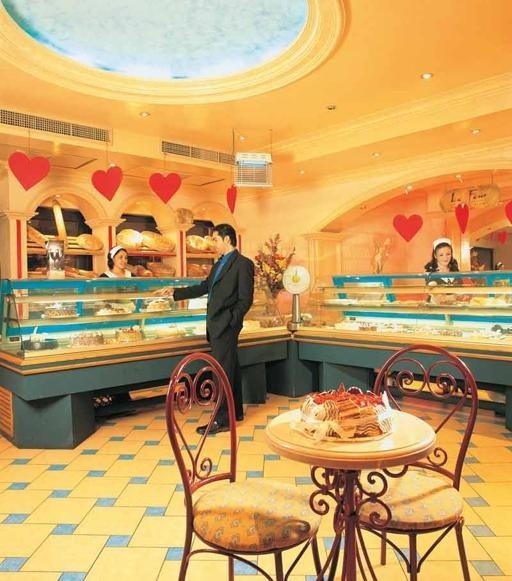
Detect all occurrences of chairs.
[344,344,478,581]
[164,353,324,581]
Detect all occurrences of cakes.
[44,305,76,317]
[297,380,396,445]
[113,324,144,341]
[137,299,171,312]
[94,304,129,316]
[21,334,59,350]
[69,331,104,347]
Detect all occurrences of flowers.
[253,233,295,298]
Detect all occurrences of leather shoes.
[197,420,231,434]
[236,416,243,421]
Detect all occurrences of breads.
[27,225,218,280]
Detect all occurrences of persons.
[423,238,472,396]
[153,224,255,436]
[95,246,147,401]
[491,259,507,287]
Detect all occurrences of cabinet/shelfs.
[293,271,512,432]
[0,278,292,449]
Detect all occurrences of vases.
[260,289,285,327]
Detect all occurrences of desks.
[262,405,437,581]
